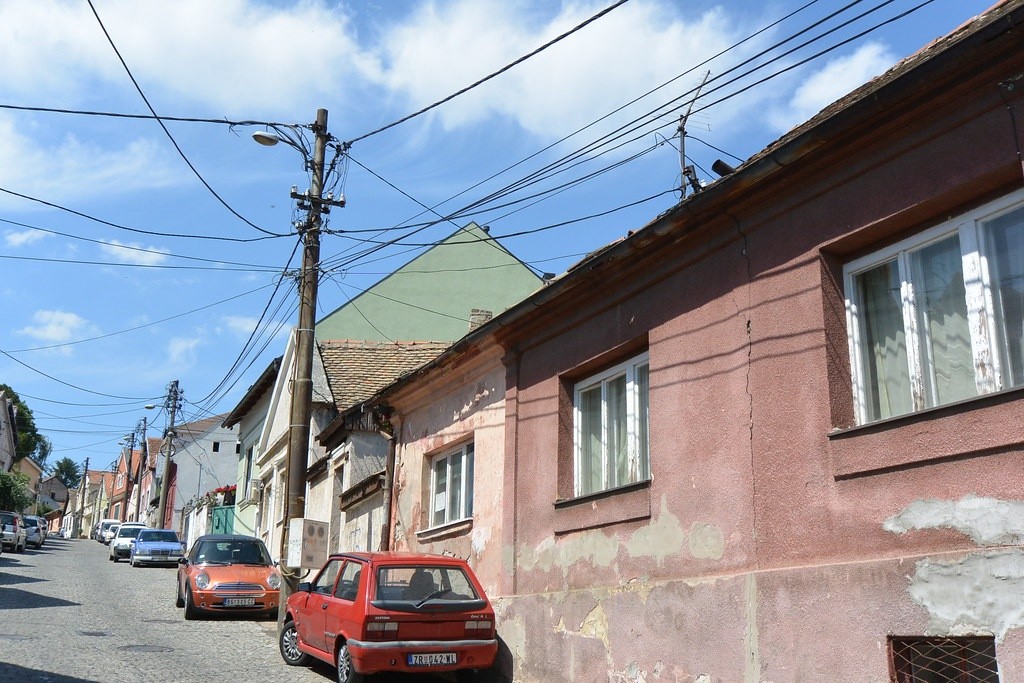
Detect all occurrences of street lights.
[143,403,176,530]
[248,130,323,635]
[118,441,134,521]
[123,436,145,522]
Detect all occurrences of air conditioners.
[34,483,42,490]
[34,493,40,502]
[245,479,263,505]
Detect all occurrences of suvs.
[0,510,30,553]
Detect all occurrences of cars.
[22,515,47,549]
[109,525,152,563]
[90,519,147,545]
[174,534,282,620]
[40,518,49,536]
[279,552,499,683]
[129,529,186,567]
